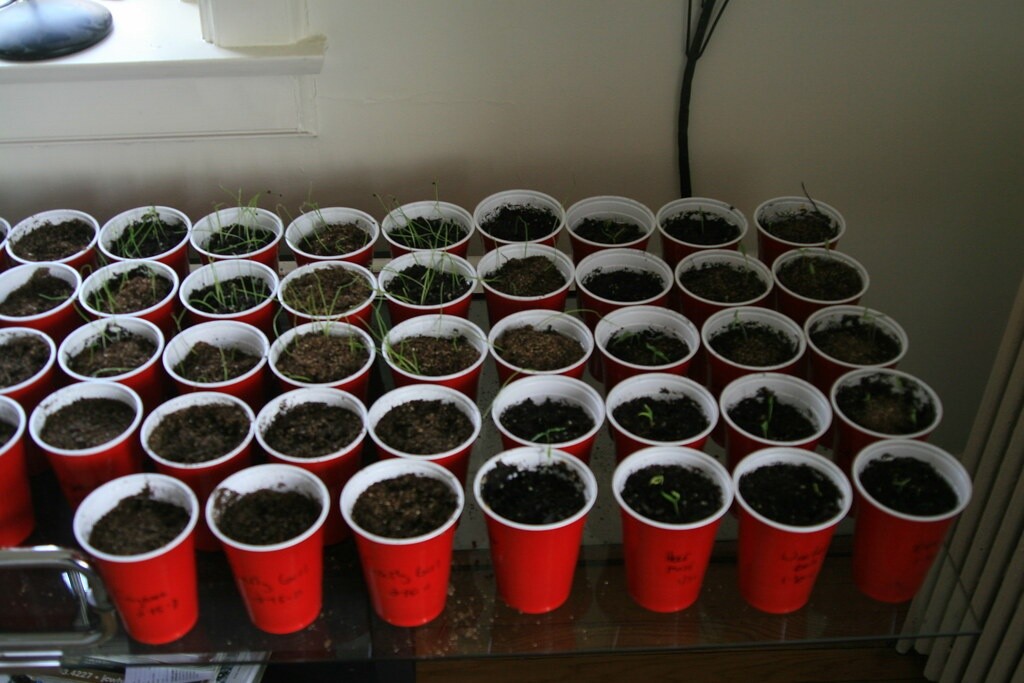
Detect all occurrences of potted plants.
[2,186,972,647]
